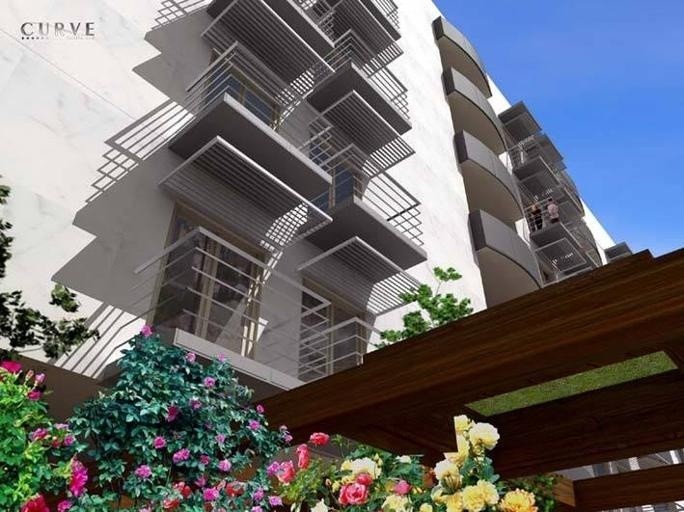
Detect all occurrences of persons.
[532,202,544,230]
[547,197,560,223]
